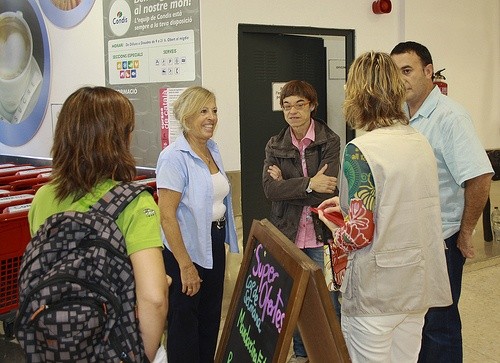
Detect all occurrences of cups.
[0,11,33,112]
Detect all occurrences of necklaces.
[202,151,210,165]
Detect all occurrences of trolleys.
[0,162,158,341]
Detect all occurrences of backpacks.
[11,180,157,363]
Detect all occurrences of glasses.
[281,101,312,111]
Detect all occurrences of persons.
[28,86,172,363]
[389,41,495,363]
[317,49,453,363]
[259,80,341,363]
[156,87,240,363]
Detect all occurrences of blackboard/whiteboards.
[214,218,352,363]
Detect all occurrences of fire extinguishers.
[431,68,447,95]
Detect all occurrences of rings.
[187,289,193,291]
